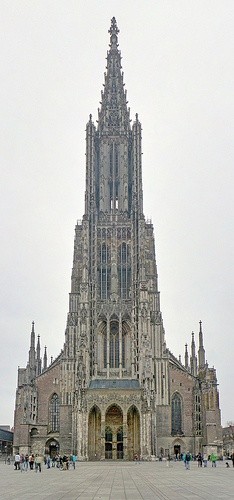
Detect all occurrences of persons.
[132,451,234,471]
[0,450,78,472]
